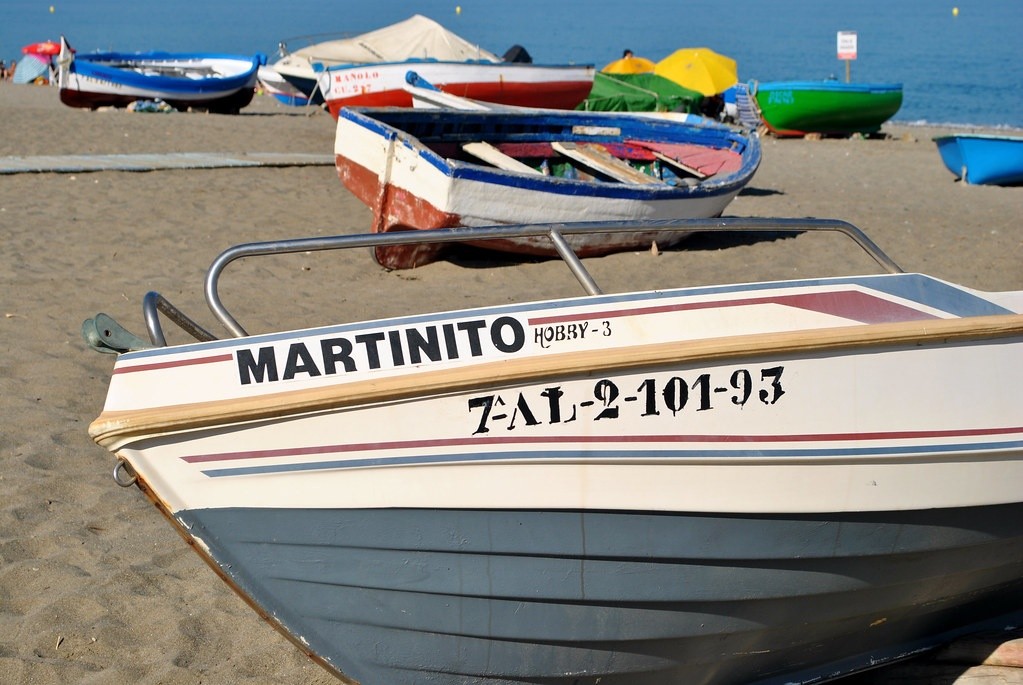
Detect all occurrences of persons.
[623,49,635,57]
[0,59,8,81]
[8,60,17,75]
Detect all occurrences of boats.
[747,75,903,140]
[271,15,533,111]
[81,217,1023,685]
[311,58,597,124]
[931,135,1023,185]
[58,35,267,114]
[334,105,761,271]
[403,70,730,125]
[256,63,315,106]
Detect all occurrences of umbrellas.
[12,39,76,83]
[600,57,659,75]
[651,46,742,96]
